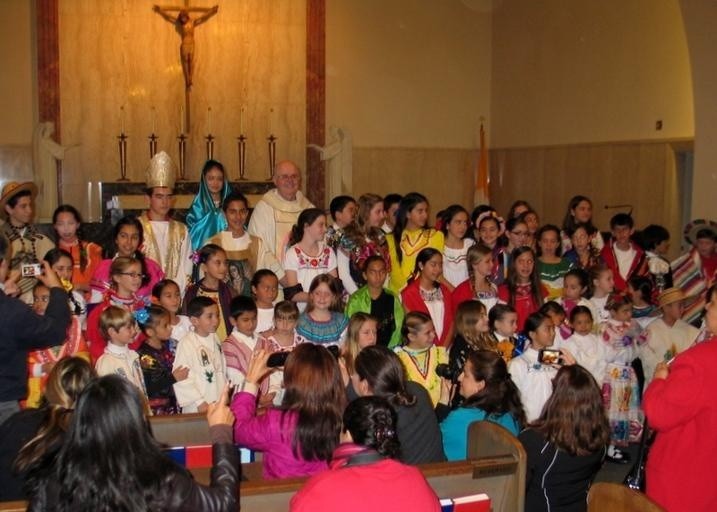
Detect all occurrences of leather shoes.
[606,448,632,465]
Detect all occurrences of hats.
[1,182,39,221]
[146,150,176,189]
[658,288,689,310]
[684,219,717,245]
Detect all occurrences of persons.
[152,3,219,91]
[1,160,717,511]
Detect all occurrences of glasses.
[118,272,144,278]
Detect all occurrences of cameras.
[268,353,286,366]
[435,362,462,383]
[21,262,42,278]
[537,348,561,364]
